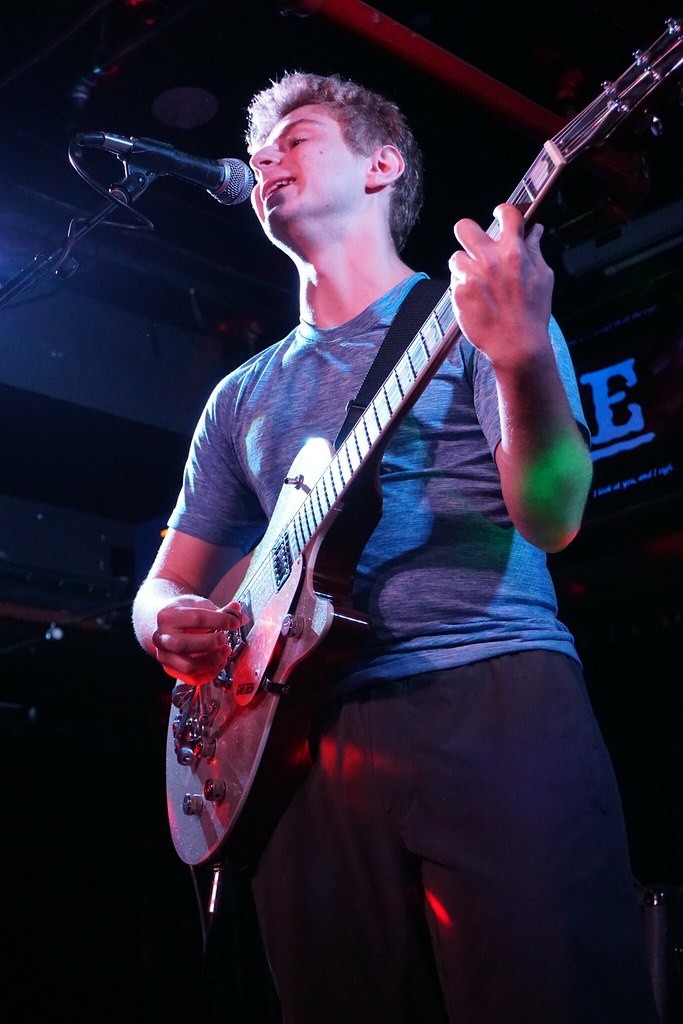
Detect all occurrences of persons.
[134,68,647,1024]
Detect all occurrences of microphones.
[76,131,254,205]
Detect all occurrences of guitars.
[165,20,683,869]
